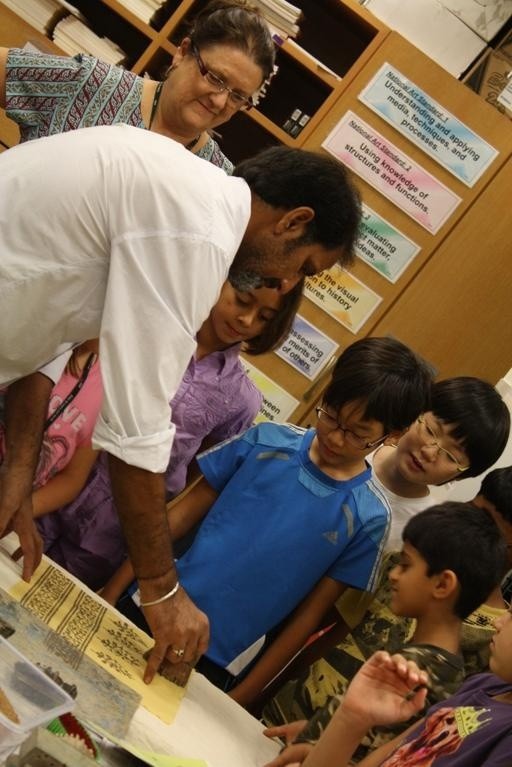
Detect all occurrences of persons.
[1,0,511,767]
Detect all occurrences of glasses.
[188,39,254,113]
[416,412,472,474]
[314,393,391,451]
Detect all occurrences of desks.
[0,517,294,767]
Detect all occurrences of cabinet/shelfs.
[2,0,512,438]
